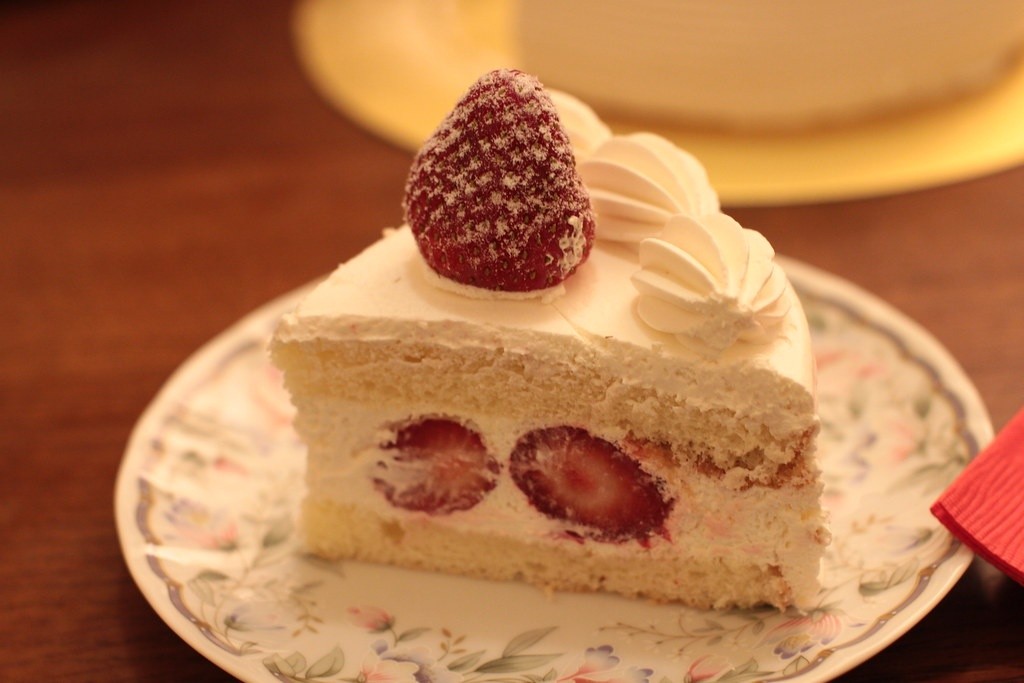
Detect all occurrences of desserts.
[508,0,1023,133]
[270,70,832,613]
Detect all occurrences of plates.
[293,0,1023,210]
[112,251,994,683]
